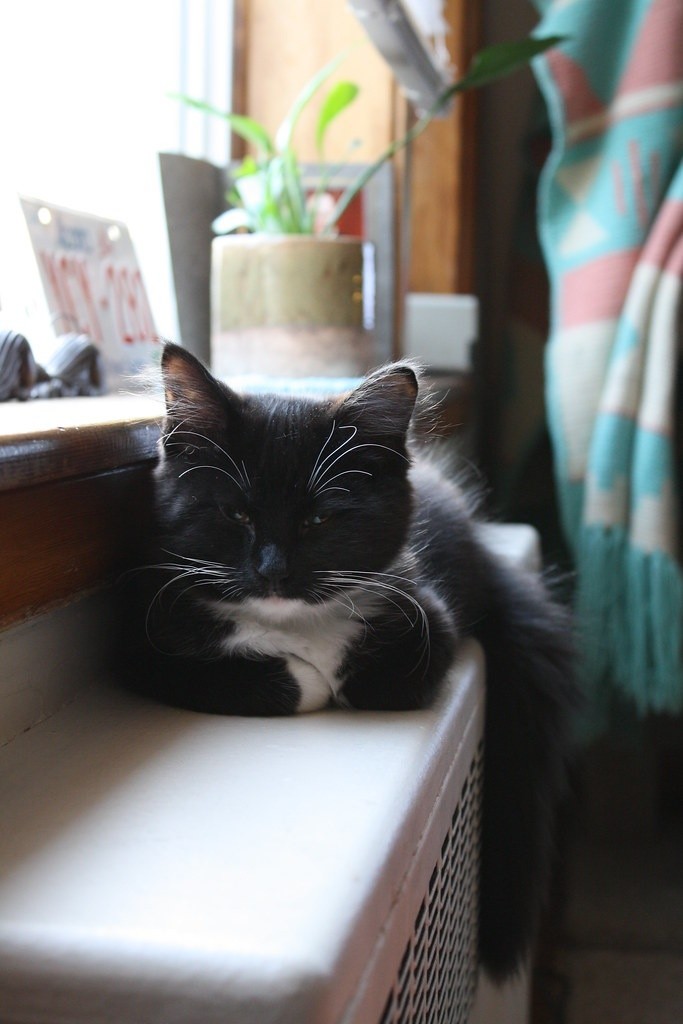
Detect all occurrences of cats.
[114,333,591,991]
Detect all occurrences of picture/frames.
[222,158,394,365]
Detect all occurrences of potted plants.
[168,30,574,373]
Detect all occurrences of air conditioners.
[0,524,548,1024]
